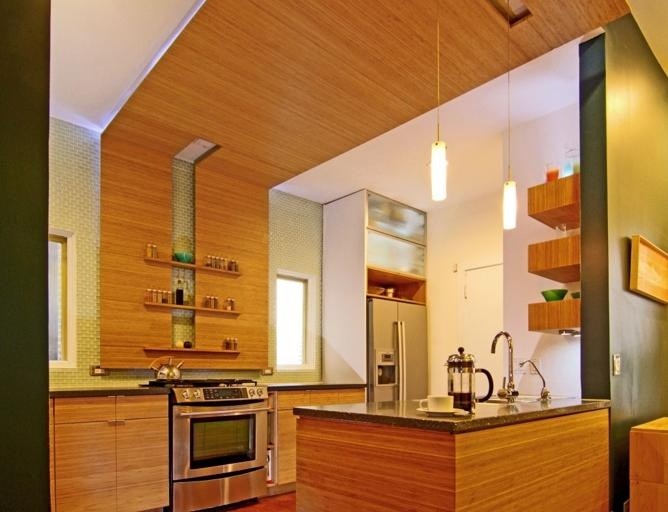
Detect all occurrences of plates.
[416,408,468,417]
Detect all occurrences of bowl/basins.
[541,289,568,301]
[570,292,580,299]
[368,286,385,296]
[174,252,193,264]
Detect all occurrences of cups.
[553,223,566,239]
[545,159,580,182]
[385,288,393,298]
[419,395,454,411]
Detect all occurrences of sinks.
[513,395,541,403]
[483,398,526,406]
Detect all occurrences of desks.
[628,415,667,512]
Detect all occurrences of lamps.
[497,0,521,234]
[428,0,452,203]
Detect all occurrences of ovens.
[171,402,270,512]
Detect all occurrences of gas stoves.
[138,377,271,403]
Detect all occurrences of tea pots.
[147,356,184,379]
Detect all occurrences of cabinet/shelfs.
[142,253,244,357]
[274,389,364,484]
[522,172,580,338]
[321,189,429,303]
[52,394,170,512]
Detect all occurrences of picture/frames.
[627,232,667,306]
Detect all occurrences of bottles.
[146,242,158,258]
[446,347,495,413]
[223,337,238,350]
[206,256,239,270]
[143,288,173,304]
[175,280,191,306]
[204,295,235,311]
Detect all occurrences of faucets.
[519,360,550,403]
[490,331,519,404]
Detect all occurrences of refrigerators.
[368,297,429,402]
